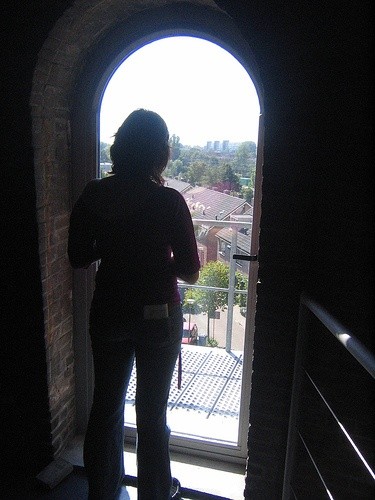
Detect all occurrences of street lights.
[186,299,195,344]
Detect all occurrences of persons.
[66,108,202,500]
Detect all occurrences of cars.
[181,321,198,344]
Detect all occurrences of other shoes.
[167,477,181,500]
